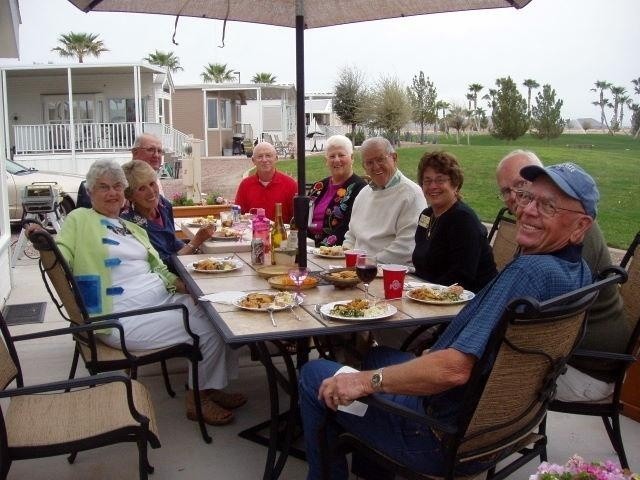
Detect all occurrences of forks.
[286,304,304,322]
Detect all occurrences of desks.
[233,137,244,154]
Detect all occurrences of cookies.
[330,269,357,282]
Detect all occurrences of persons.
[25,159,249,426]
[412,152,499,295]
[296,162,601,480]
[235,142,298,224]
[131,132,174,230]
[119,159,217,276]
[341,136,429,274]
[496,150,631,404]
[306,135,368,248]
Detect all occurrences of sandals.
[187,400,235,424]
[209,390,248,410]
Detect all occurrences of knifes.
[292,294,305,318]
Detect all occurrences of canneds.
[231,204,242,224]
[250,237,265,266]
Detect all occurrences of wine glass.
[289,267,309,298]
[357,255,376,302]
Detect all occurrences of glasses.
[516,191,587,215]
[136,147,164,155]
[363,156,392,169]
[422,176,451,184]
[498,180,530,202]
[93,185,123,191]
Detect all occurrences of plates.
[377,264,416,277]
[211,231,239,239]
[269,275,324,289]
[189,259,243,273]
[232,292,303,312]
[313,248,367,258]
[317,268,362,288]
[320,299,398,320]
[257,264,295,277]
[187,219,216,226]
[407,285,476,304]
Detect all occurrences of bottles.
[289,193,299,261]
[231,205,241,223]
[271,203,287,265]
[250,207,270,265]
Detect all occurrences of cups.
[201,193,208,207]
[273,249,297,265]
[219,212,233,227]
[315,234,321,248]
[345,249,366,268]
[383,264,407,300]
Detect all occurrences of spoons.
[315,303,329,324]
[267,306,278,327]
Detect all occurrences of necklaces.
[427,199,457,238]
[109,217,128,236]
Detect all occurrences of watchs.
[187,243,199,254]
[370,367,385,394]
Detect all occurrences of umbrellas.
[68,0,533,370]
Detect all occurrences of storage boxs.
[223,149,233,156]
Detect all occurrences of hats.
[520,162,599,219]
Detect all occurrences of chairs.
[262,132,291,158]
[534,228,640,471]
[77,181,92,208]
[27,225,212,462]
[0,312,161,480]
[326,265,629,480]
[61,195,75,213]
[489,208,522,271]
